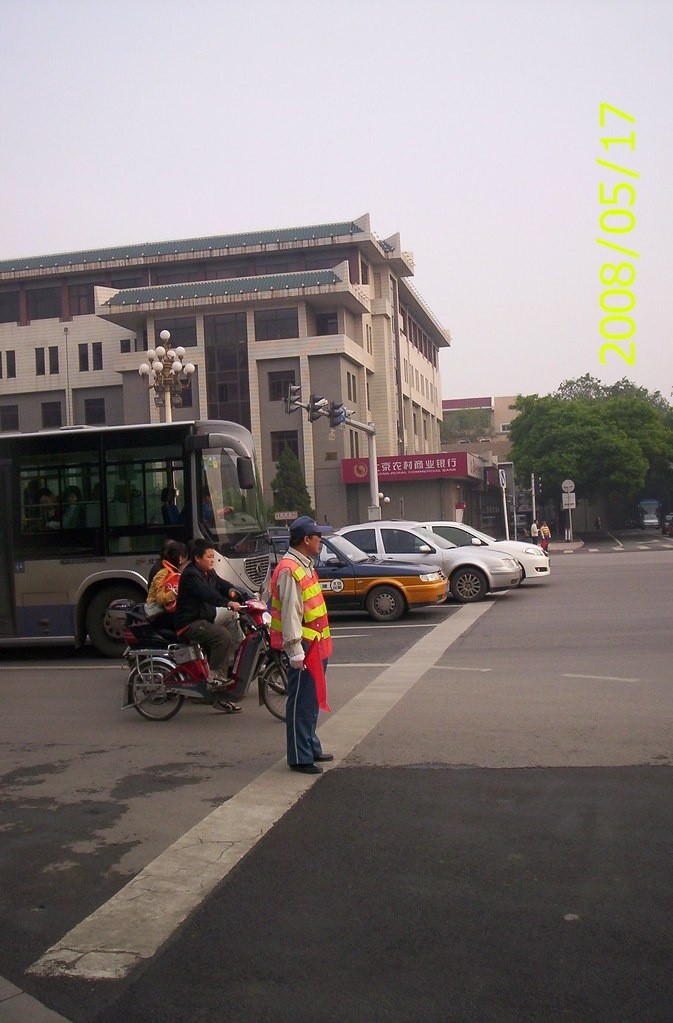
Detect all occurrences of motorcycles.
[120,584,290,721]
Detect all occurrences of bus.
[636,500,662,517]
[0,419,279,659]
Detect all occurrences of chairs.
[158,487,180,523]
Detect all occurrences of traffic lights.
[330,402,344,427]
[309,394,324,422]
[285,383,301,414]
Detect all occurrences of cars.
[417,520,552,588]
[267,533,450,623]
[640,514,660,531]
[319,520,522,603]
[659,513,673,535]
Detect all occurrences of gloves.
[290,653,306,671]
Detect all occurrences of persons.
[174,538,243,711]
[540,521,551,552]
[270,516,333,774]
[179,485,234,524]
[24,480,82,529]
[144,539,187,631]
[531,520,539,545]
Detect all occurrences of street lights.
[137,329,195,504]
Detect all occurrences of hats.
[290,516,332,537]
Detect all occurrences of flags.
[303,636,331,712]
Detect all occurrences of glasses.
[311,533,321,538]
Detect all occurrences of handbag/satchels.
[162,560,182,612]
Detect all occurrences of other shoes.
[289,763,323,774]
[207,676,234,686]
[314,754,333,761]
[212,701,242,712]
